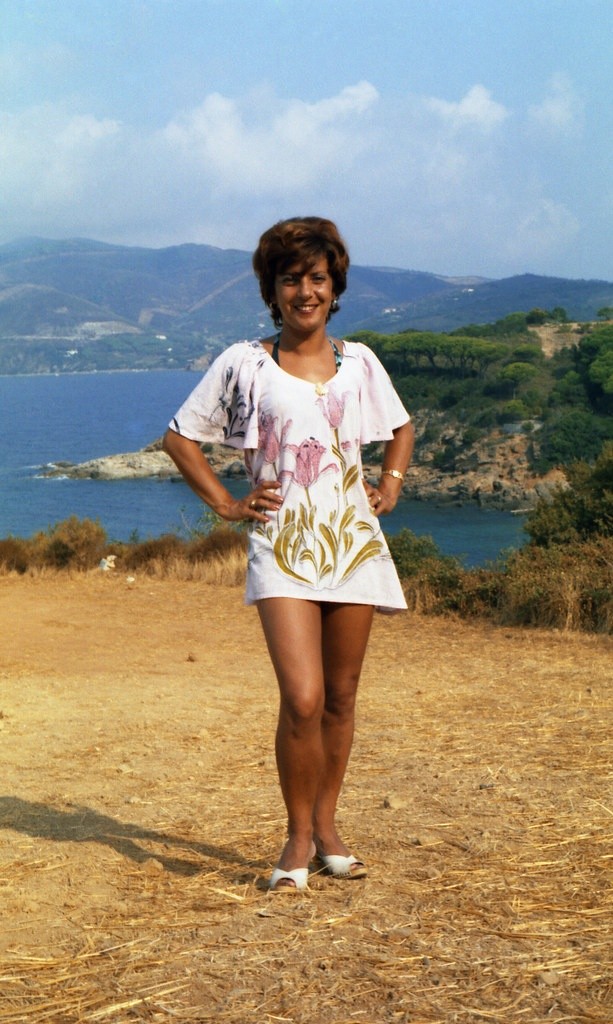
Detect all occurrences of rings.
[251,499,256,508]
[376,496,382,503]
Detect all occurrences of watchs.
[382,469,404,484]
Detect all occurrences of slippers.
[269,868,308,894]
[317,848,369,879]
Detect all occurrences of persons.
[161,216,417,889]
